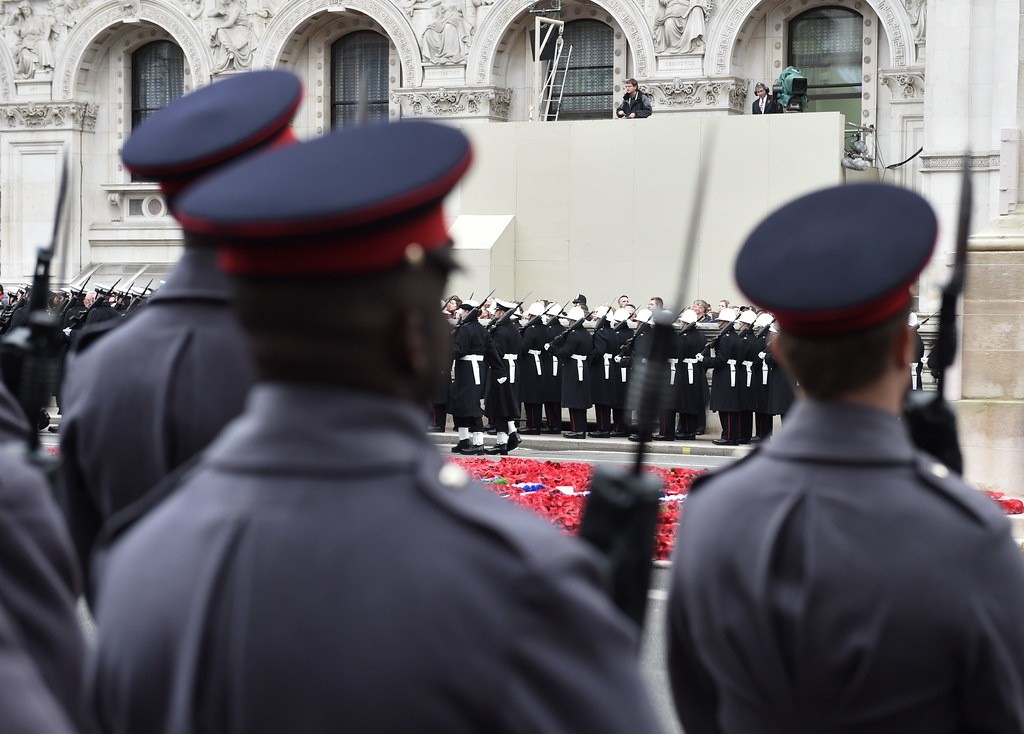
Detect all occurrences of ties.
[760,98,764,112]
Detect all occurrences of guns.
[0,260,172,337]
[0,145,73,444]
[890,145,976,486]
[440,286,942,356]
[575,116,717,660]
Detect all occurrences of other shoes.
[39,410,50,430]
[48,423,60,433]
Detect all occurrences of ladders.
[538,44,573,120]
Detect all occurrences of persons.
[662,183,1024,734]
[58,67,309,560]
[752,83,784,114]
[0,382,95,734]
[89,117,660,734]
[1,286,923,446]
[615,78,653,119]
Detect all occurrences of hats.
[493,299,516,310]
[119,68,306,189]
[572,294,586,304]
[6,279,167,301]
[177,114,471,283]
[731,180,941,339]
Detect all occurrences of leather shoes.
[461,444,484,455]
[711,436,769,445]
[427,421,706,443]
[507,431,522,452]
[486,444,509,455]
[450,440,471,452]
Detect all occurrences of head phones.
[754,83,770,96]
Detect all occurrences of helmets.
[440,299,777,333]
[908,311,919,326]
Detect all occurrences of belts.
[459,350,769,387]
[908,363,919,390]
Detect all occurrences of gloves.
[63,327,72,336]
[696,353,704,362]
[758,351,766,359]
[544,343,550,350]
[497,377,508,384]
[614,355,621,363]
